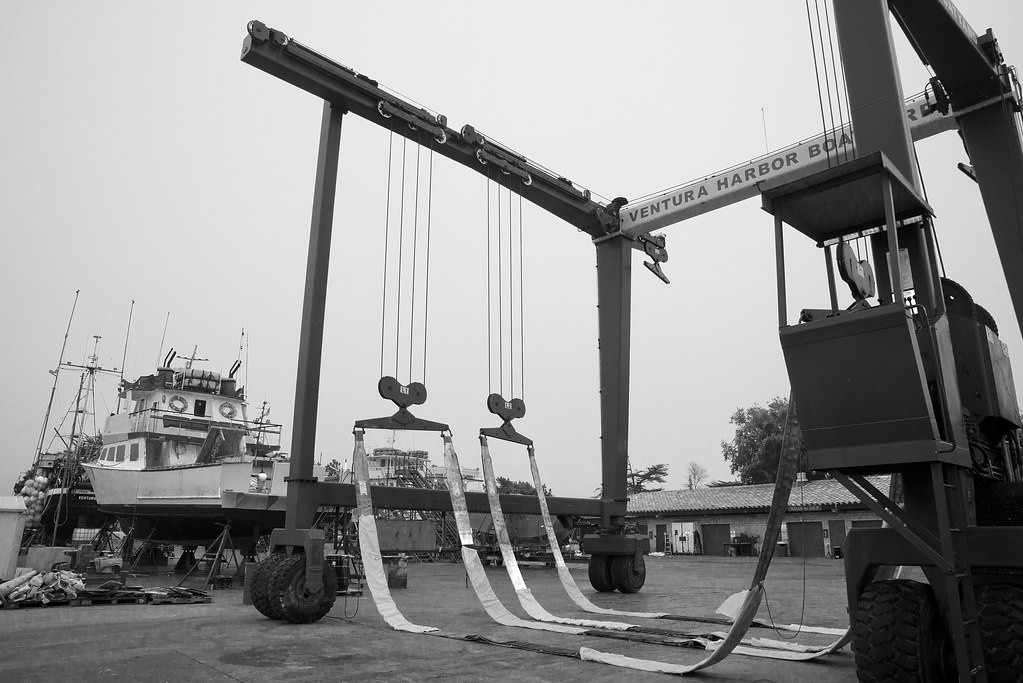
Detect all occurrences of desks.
[724,543,754,557]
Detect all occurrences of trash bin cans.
[325,554,350,591]
[833,546,842,559]
[777,542,787,557]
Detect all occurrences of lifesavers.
[219,403,237,418]
[182,378,221,391]
[175,372,183,381]
[174,381,182,388]
[169,395,188,412]
[182,369,220,381]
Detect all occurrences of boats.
[36,288,353,551]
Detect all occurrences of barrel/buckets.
[325,554,351,592]
[386,559,407,589]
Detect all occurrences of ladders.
[664,534,672,555]
